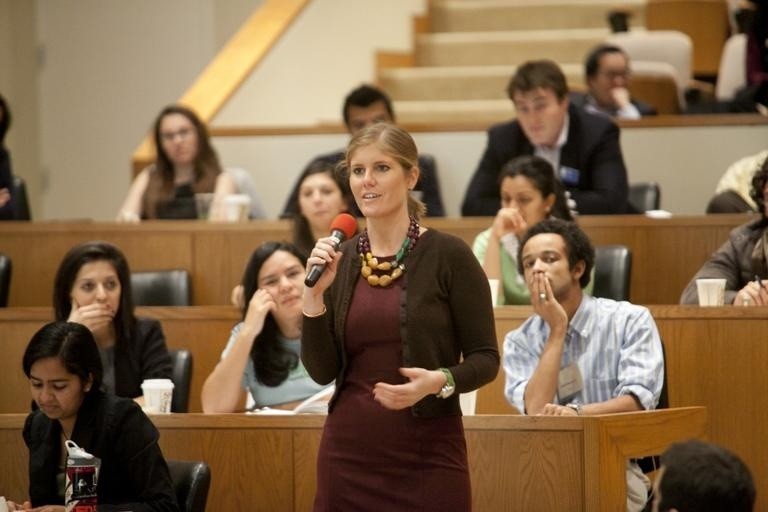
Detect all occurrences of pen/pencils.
[755,274,763,288]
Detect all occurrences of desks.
[1,305,756,466]
[0,209,766,302]
[0,407,707,512]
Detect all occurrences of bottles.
[64,440,101,512]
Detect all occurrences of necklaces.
[59,432,71,444]
[358,216,419,286]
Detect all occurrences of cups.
[141,379,175,414]
[695,278,727,307]
[225,195,250,221]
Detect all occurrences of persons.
[114,105,267,221]
[290,161,365,268]
[653,437,756,511]
[679,155,768,307]
[461,59,629,220]
[281,85,445,217]
[571,47,658,116]
[52,243,176,415]
[6,321,175,512]
[471,157,596,302]
[0,93,29,223]
[502,220,665,510]
[200,241,336,413]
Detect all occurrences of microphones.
[304,214,357,289]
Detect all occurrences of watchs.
[566,404,584,416]
[438,368,454,398]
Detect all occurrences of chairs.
[626,183,661,213]
[128,269,192,306]
[164,459,210,511]
[589,245,632,303]
[165,348,192,413]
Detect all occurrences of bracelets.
[304,305,327,318]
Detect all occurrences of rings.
[540,294,546,299]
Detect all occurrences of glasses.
[595,68,630,80]
